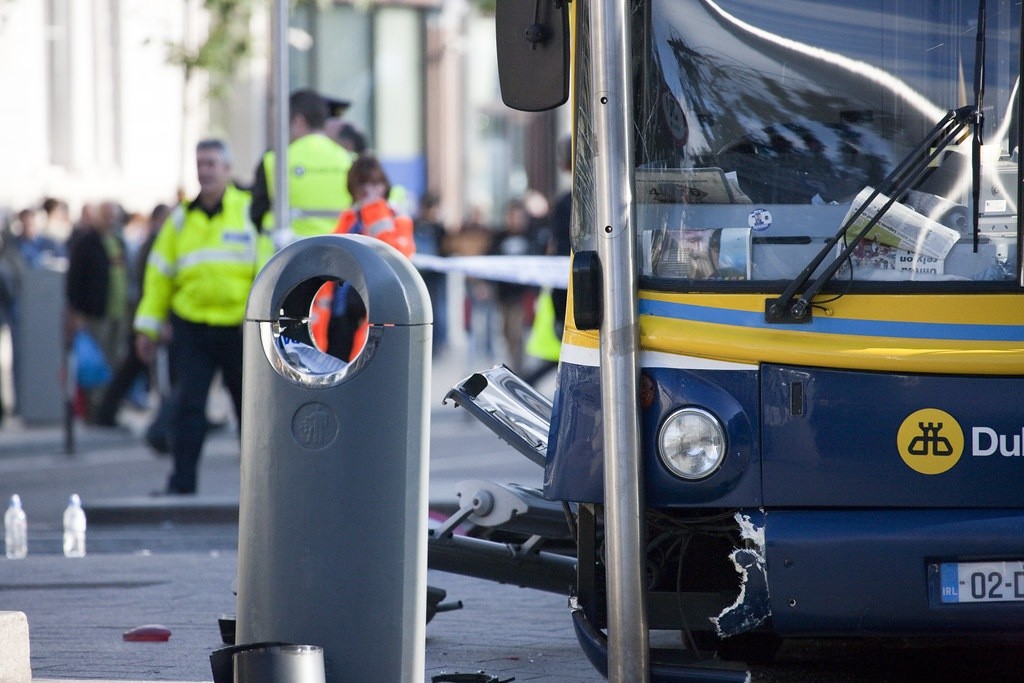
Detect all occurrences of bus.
[427,0,1021,683]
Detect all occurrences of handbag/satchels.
[69,332,111,389]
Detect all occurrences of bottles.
[61,493,87,559]
[4,493,28,560]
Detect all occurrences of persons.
[308,159,417,359]
[445,136,573,383]
[412,189,447,355]
[133,138,274,493]
[251,92,409,285]
[1,184,228,451]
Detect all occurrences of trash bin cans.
[232,234,436,683]
